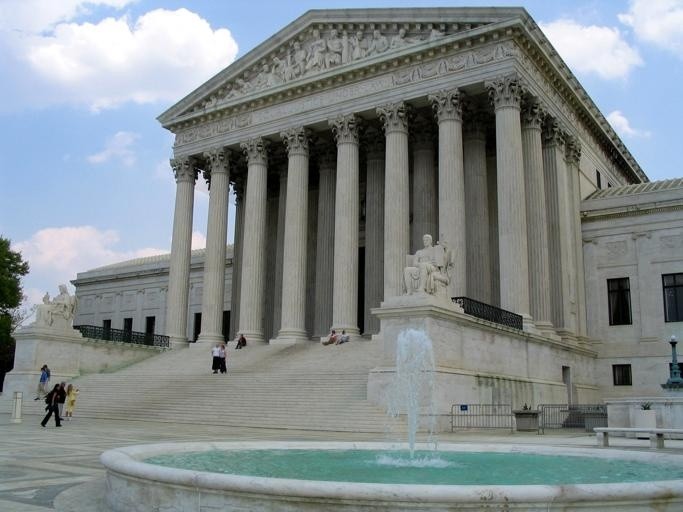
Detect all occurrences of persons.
[402,232,444,296]
[224,28,421,100]
[322,330,337,346]
[211,344,220,374]
[234,334,246,349]
[30,284,71,326]
[33,364,81,427]
[219,345,227,375]
[335,330,349,346]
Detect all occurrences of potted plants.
[511,403,540,431]
[635,401,663,438]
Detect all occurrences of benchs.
[593,427,683,448]
